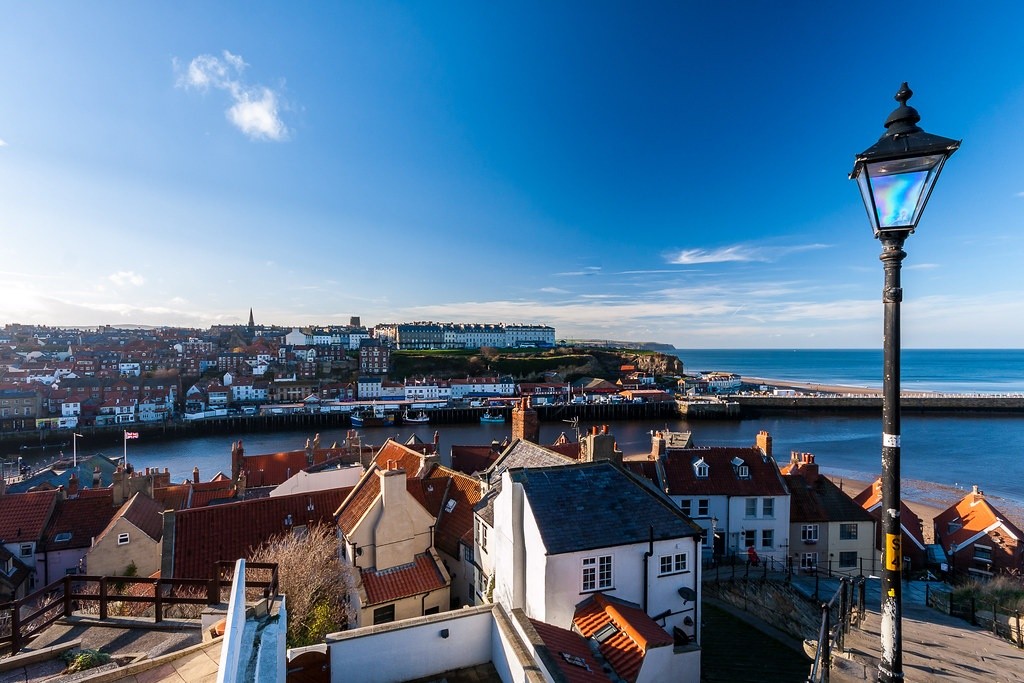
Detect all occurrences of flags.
[126,431,139,440]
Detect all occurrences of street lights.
[848,80,962,682]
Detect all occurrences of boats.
[2,456,30,475]
[481,409,506,424]
[349,399,430,429]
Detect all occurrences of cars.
[733,390,775,397]
[227,406,260,416]
[287,385,712,415]
